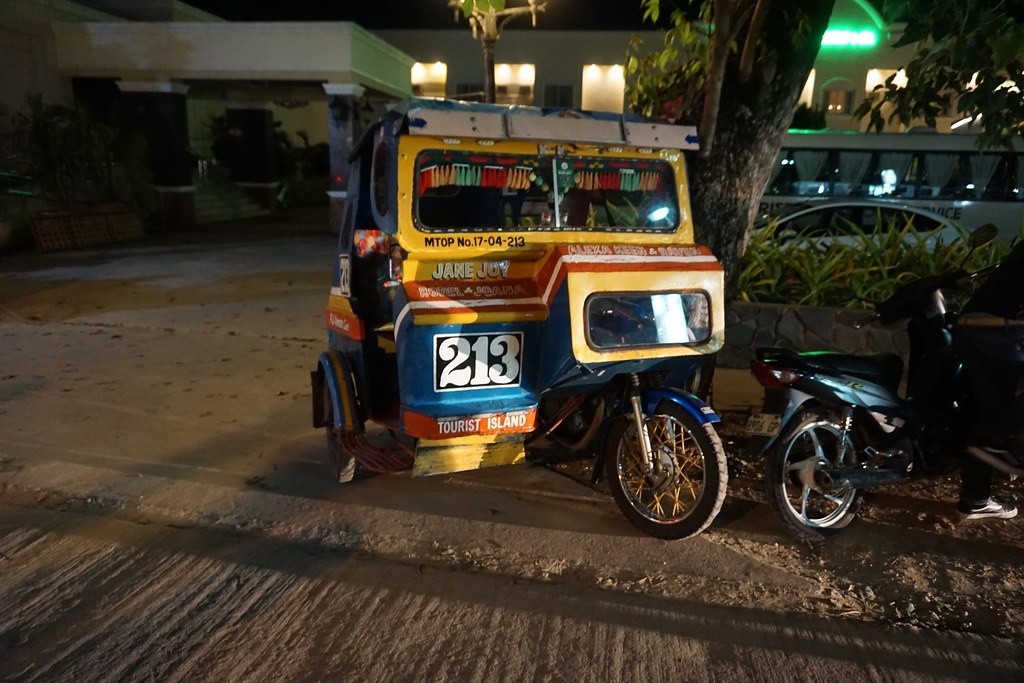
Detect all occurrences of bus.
[752,133,1023,261]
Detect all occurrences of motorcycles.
[748,223,1024,538]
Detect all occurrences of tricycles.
[310,97,728,543]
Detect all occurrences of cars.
[754,200,968,255]
